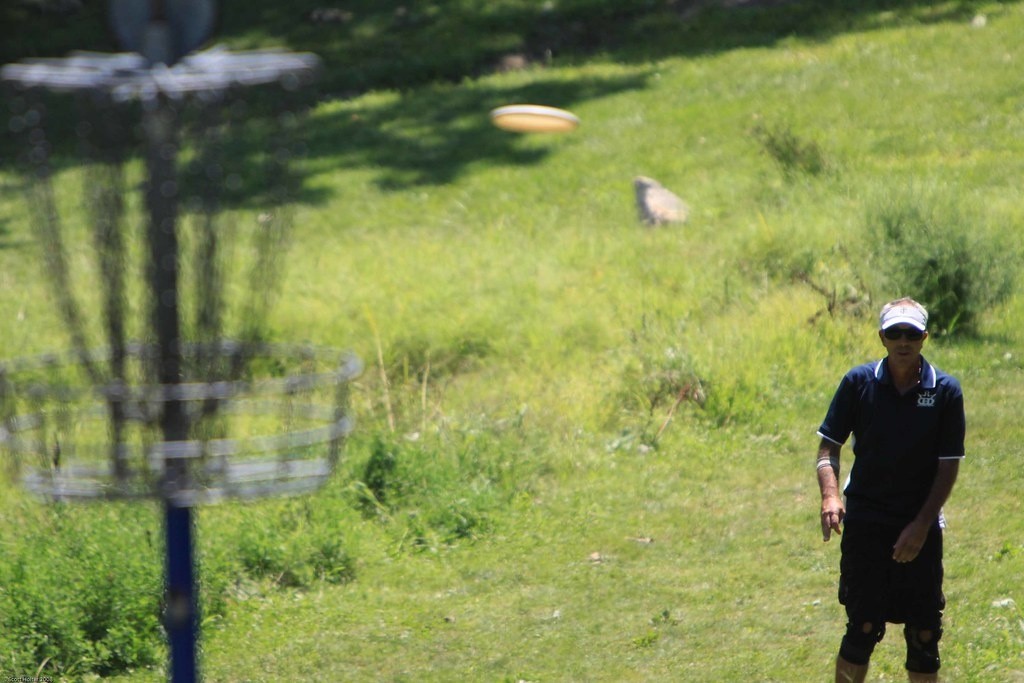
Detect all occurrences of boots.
[908,671,937,683]
[835,655,868,683]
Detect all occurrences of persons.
[814,296,967,683]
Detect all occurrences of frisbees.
[490,102,580,135]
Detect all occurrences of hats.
[880,297,928,331]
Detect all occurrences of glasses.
[885,326,924,341]
